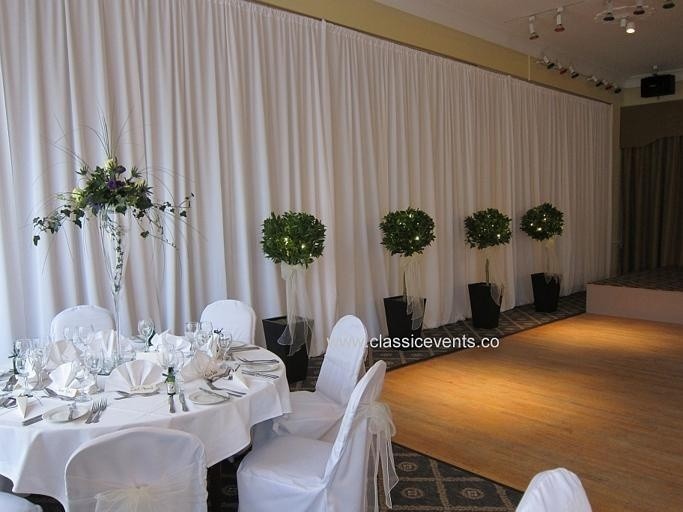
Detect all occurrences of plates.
[42,404,89,421]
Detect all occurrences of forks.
[85,403,97,425]
[92,398,106,423]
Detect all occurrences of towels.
[179,349,211,384]
[149,329,192,355]
[45,361,82,398]
[103,359,164,397]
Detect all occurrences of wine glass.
[12,318,282,405]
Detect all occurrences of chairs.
[515,466,593,512]
[237,360,386,512]
[268,314,369,442]
[49,304,117,363]
[200,299,258,345]
[1,489,44,512]
[64,425,207,512]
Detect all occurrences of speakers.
[640,74,676,97]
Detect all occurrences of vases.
[97,209,131,366]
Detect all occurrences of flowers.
[22,85,209,298]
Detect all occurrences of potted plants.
[377,207,437,350]
[518,203,565,314]
[259,206,327,383]
[464,207,514,330]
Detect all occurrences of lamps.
[525,0,677,41]
[536,55,623,95]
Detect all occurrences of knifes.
[167,394,175,412]
[178,393,189,411]
